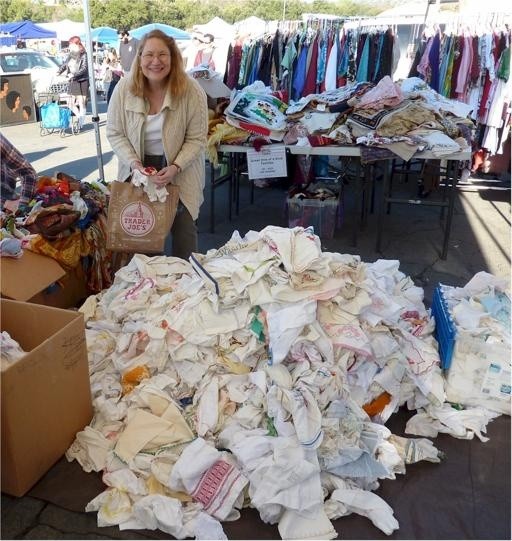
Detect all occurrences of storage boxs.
[197,78,231,110]
[0,248,87,309]
[286,183,342,243]
[0,298,94,498]
[445,285,511,415]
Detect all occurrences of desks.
[209,145,375,247]
[375,147,472,260]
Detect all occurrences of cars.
[0,45,105,114]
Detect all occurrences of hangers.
[238,16,512,34]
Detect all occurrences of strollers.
[38,73,72,139]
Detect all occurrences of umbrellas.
[91,26,118,64]
[128,22,190,40]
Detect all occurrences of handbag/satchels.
[106,180,179,254]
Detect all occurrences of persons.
[1,134,36,228]
[16,38,57,56]
[6,91,21,113]
[105,31,208,280]
[22,106,31,121]
[56,36,88,130]
[117,28,139,75]
[194,34,215,70]
[93,44,116,96]
[0,78,10,99]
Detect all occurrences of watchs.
[172,163,182,173]
